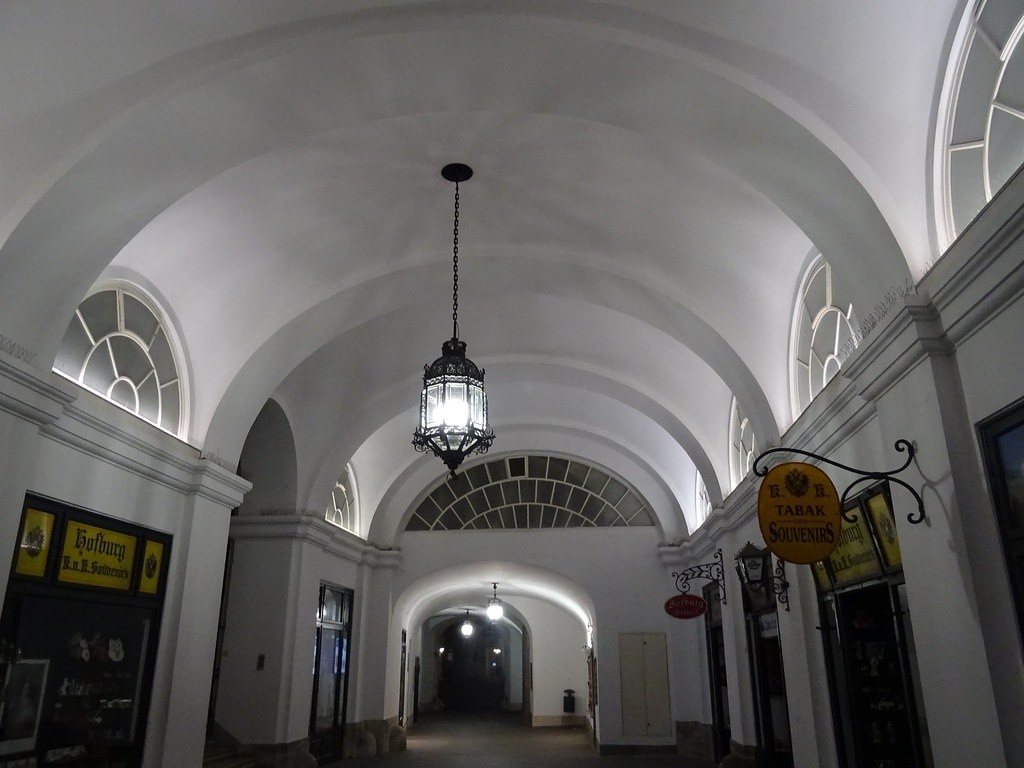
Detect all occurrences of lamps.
[487,582,503,624]
[461,609,473,639]
[412,163,496,479]
